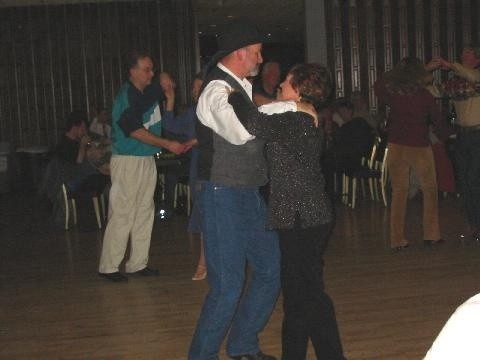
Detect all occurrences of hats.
[209,21,267,65]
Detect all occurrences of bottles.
[158,188,170,222]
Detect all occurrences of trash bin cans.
[0,142,11,194]
[17,146,50,193]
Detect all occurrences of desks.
[16,145,50,186]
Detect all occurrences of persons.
[47,106,112,196]
[317,97,374,194]
[224,60,349,360]
[97,52,183,282]
[252,61,280,99]
[157,71,214,282]
[370,56,447,252]
[422,41,480,242]
[186,16,323,360]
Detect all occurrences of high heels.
[192,265,207,281]
[424,238,445,247]
[391,242,412,252]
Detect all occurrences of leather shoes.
[229,350,278,360]
[98,272,128,282]
[126,266,159,276]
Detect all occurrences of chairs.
[159,173,190,217]
[61,183,106,232]
[332,136,389,209]
